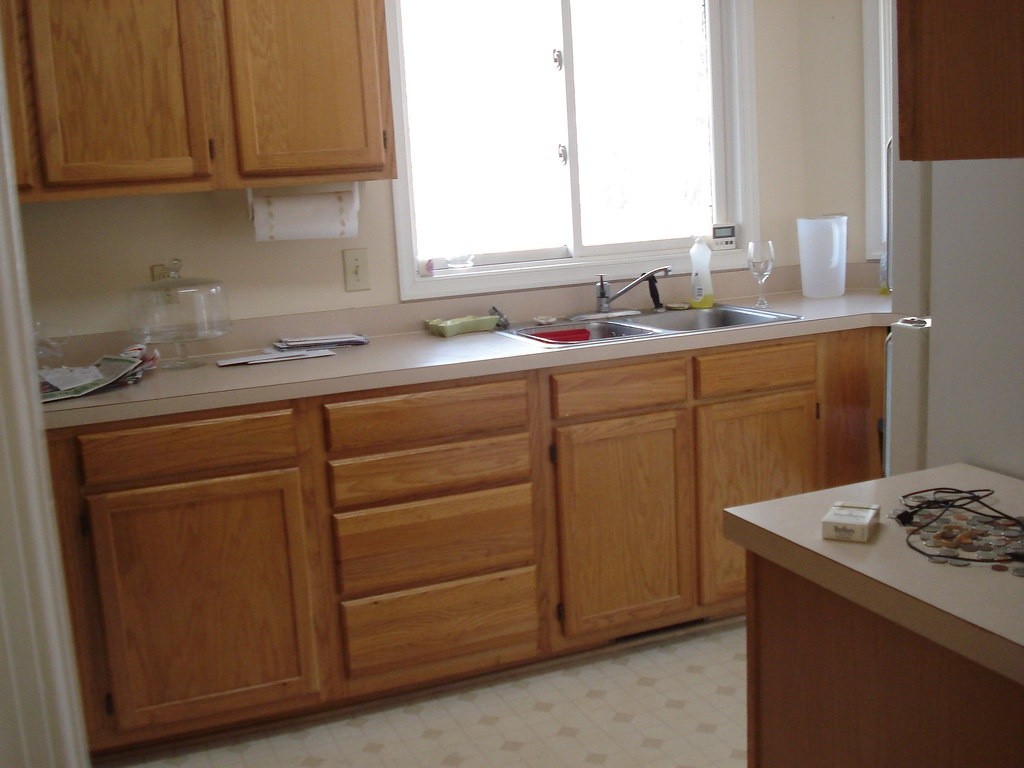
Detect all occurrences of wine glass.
[748,241,775,309]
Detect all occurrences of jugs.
[796,215,847,298]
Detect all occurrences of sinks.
[630,302,801,336]
[509,319,653,348]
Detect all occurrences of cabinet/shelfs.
[896,0,1024,161]
[310,369,552,710]
[815,325,888,489]
[539,332,822,660]
[0,0,398,203]
[744,547,1024,768]
[44,398,335,757]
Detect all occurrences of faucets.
[595,265,672,314]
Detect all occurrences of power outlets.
[150,264,186,303]
[343,248,371,291]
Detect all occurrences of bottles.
[879,241,890,295]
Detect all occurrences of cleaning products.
[689,231,715,309]
